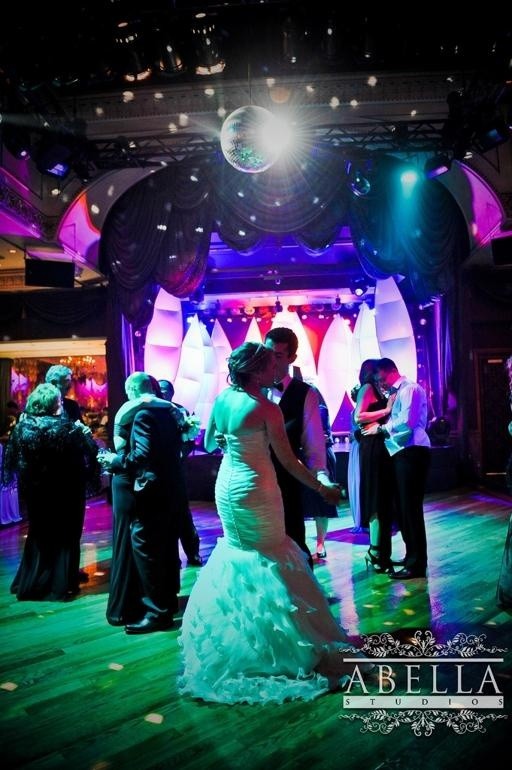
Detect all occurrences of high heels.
[364,544,395,574]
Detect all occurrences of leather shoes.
[125,611,174,634]
[389,557,426,579]
[317,551,326,558]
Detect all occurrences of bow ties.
[272,381,283,392]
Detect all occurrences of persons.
[1,327,436,707]
